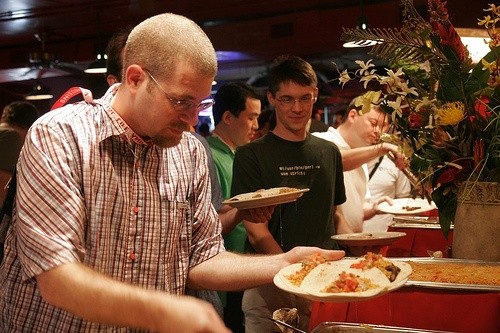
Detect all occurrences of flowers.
[327,0,500,241]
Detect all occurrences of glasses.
[273,88,317,107]
[142,67,216,112]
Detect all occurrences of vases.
[451,178,500,262]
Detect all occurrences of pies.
[300,259,413,297]
[223,186,288,202]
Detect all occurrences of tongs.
[392,217,438,225]
[386,150,417,186]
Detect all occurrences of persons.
[0,13,345,333]
[361,141,410,232]
[249,88,277,141]
[0,101,39,264]
[206,79,260,333]
[227,59,392,333]
[51,76,88,111]
[105,32,129,85]
[311,95,408,233]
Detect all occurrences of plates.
[273,258,413,301]
[374,198,437,214]
[222,189,310,210]
[331,231,406,246]
